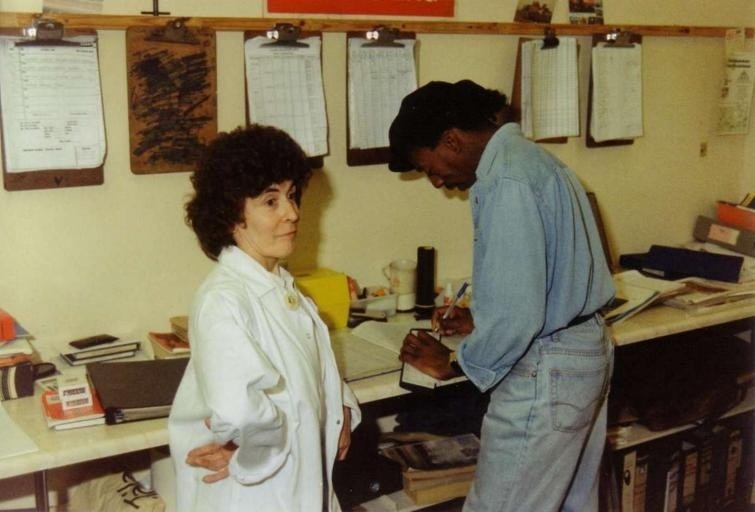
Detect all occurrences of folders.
[85,358,190,425]
[692,215,755,257]
[646,244,755,284]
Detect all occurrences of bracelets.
[448,350,461,378]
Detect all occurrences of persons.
[165,123,361,512]
[385,80,615,512]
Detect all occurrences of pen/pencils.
[434,282,469,332]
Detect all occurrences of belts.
[567,313,601,324]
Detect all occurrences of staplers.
[348,308,387,328]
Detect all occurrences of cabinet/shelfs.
[1,269,755,512]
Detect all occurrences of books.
[616,426,747,511]
[0,315,189,430]
[328,319,438,381]
[608,190,754,327]
[330,432,483,511]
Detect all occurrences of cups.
[381,259,416,312]
